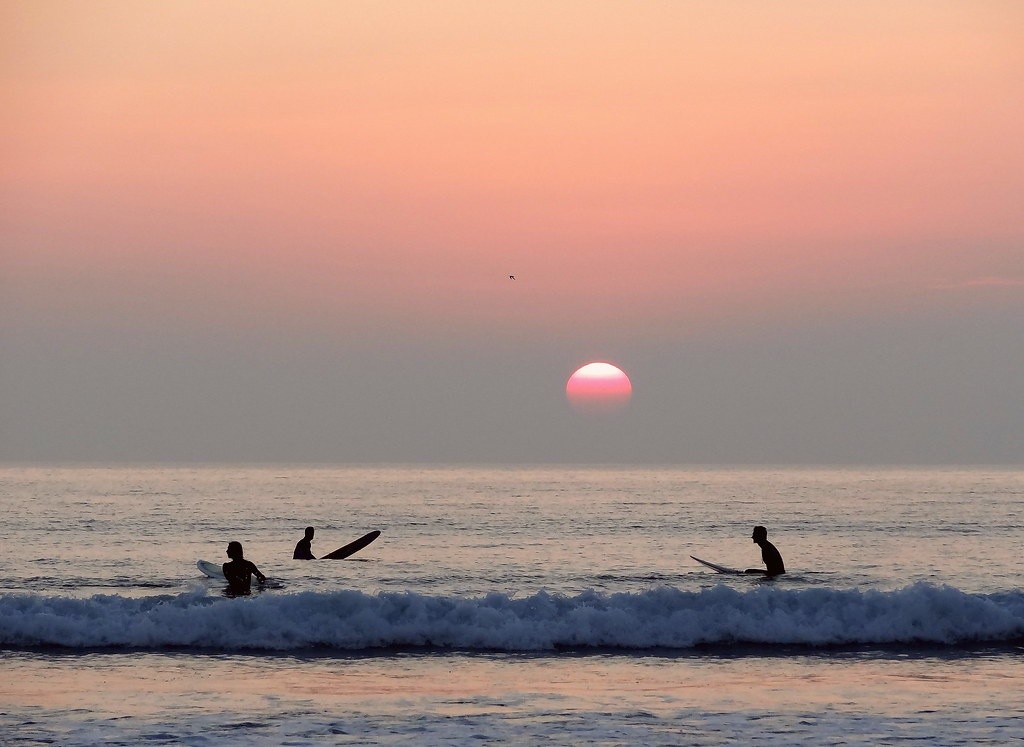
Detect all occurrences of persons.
[223,541,266,598]
[745,526,785,575]
[293,527,316,560]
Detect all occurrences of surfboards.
[688,553,749,575]
[196,559,286,590]
[320,530,383,560]
